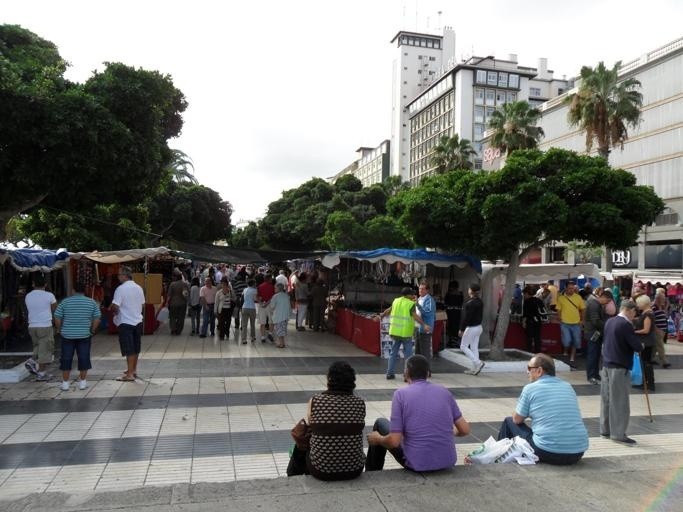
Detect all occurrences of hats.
[621,300,639,312]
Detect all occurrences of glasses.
[528,366,538,371]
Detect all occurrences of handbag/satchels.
[495,436,539,463]
[291,419,312,448]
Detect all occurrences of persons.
[598,300,644,447]
[496,353,589,467]
[374,278,486,379]
[23,266,146,391]
[286,362,367,481]
[365,354,471,474]
[497,279,681,394]
[165,262,328,348]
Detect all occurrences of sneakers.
[116,370,137,381]
[600,433,636,445]
[464,362,485,375]
[25,358,87,391]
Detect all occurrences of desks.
[333,305,356,342]
[350,313,447,358]
[105,296,166,338]
[501,314,565,358]
